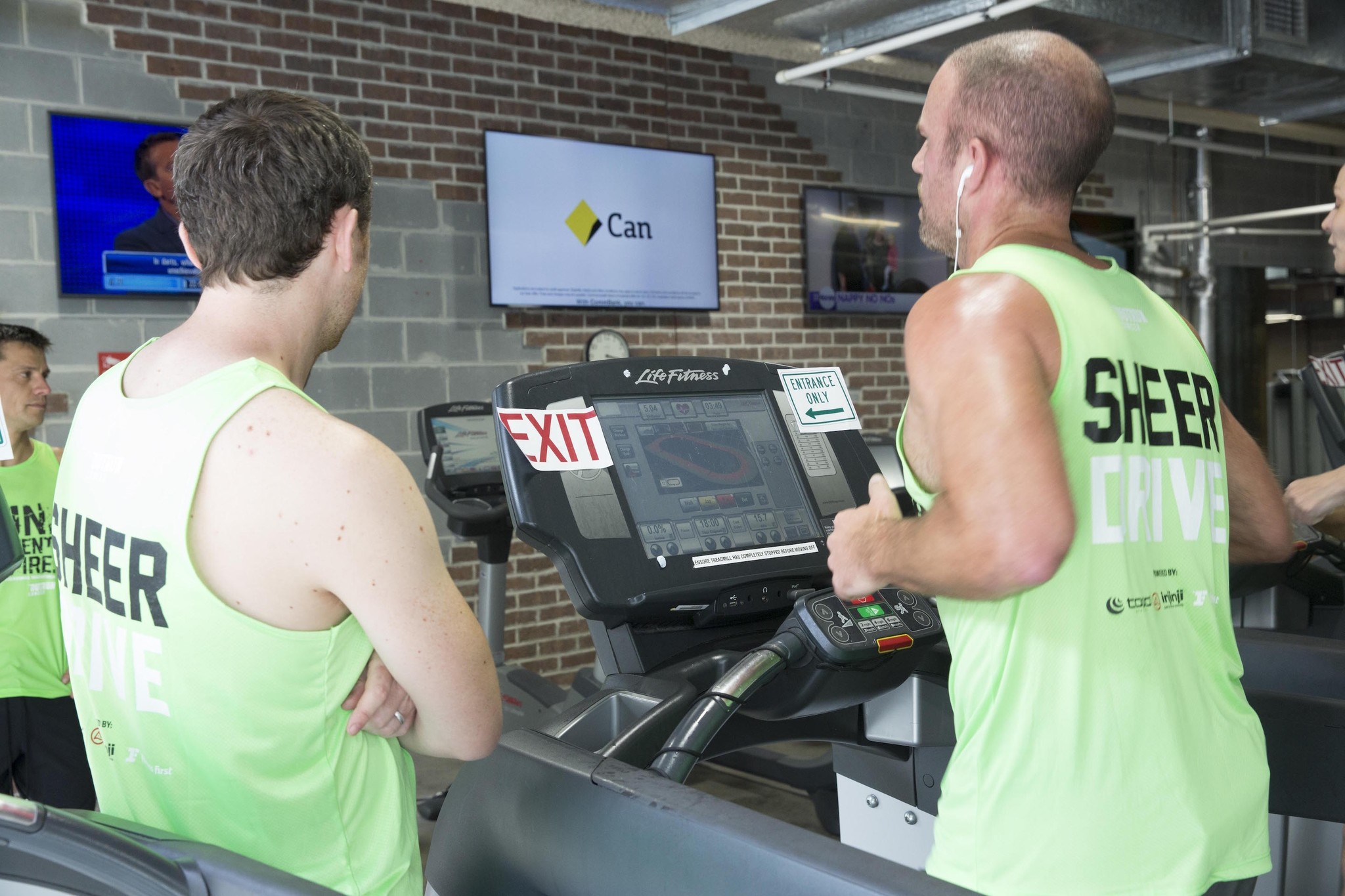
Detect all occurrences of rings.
[394,710,404,724]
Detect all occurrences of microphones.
[957,163,975,198]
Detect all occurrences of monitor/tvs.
[591,383,830,565]
[483,125,720,312]
[45,109,206,296]
[801,181,956,317]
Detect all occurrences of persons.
[0,324,96,810]
[831,206,900,292]
[115,131,186,253]
[1284,162,1345,525]
[826,31,1298,896]
[53,90,504,896]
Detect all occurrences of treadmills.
[0,794,344,896]
[418,355,1345,895]
